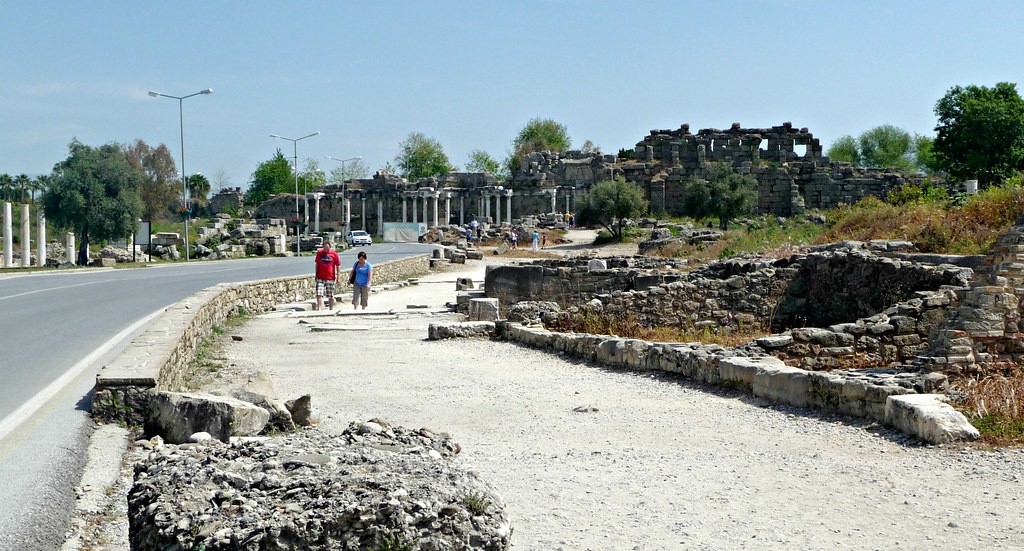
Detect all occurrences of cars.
[345,230,373,247]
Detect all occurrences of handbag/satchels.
[531,238,533,241]
[350,261,359,284]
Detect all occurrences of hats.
[534,229,538,232]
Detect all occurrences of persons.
[347,252,372,309]
[315,242,341,310]
[541,233,546,249]
[565,212,574,228]
[510,228,517,249]
[707,221,713,228]
[533,230,539,251]
[476,226,482,242]
[467,227,472,242]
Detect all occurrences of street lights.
[149,87,214,261]
[325,156,361,243]
[292,166,320,238]
[269,131,320,257]
